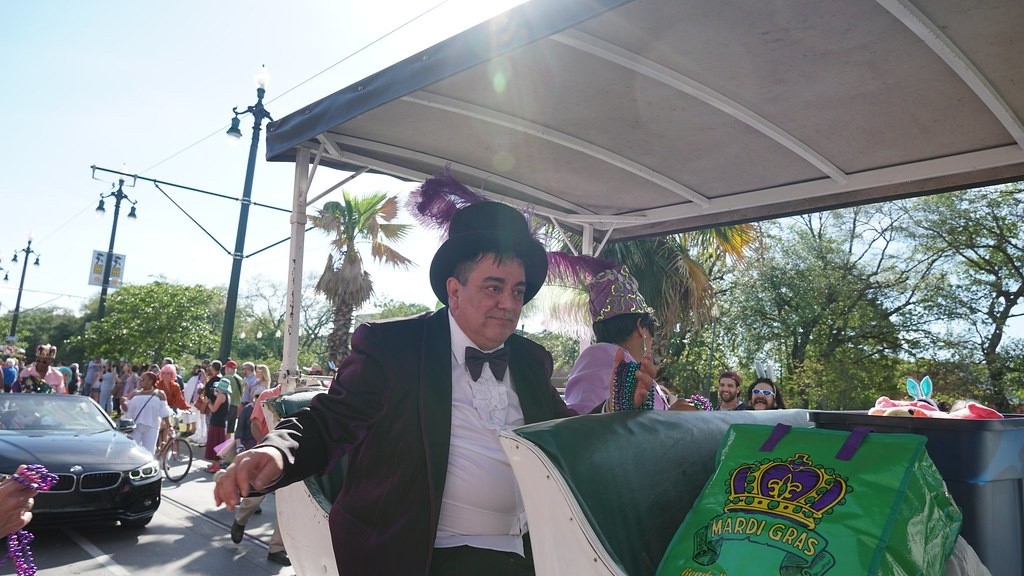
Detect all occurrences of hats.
[719,372,741,398]
[407,173,548,307]
[547,252,653,322]
[36,343,57,359]
[303,364,322,374]
[223,361,238,369]
[213,378,233,393]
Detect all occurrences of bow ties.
[465,345,510,382]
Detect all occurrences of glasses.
[36,358,51,364]
[751,389,775,396]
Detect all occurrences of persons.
[746,361,784,410]
[714,372,748,411]
[59,355,271,472]
[0,344,66,394]
[231,383,291,567]
[0,464,39,539]
[565,266,698,417]
[215,202,657,576]
[303,361,338,376]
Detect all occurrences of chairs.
[2,408,41,428]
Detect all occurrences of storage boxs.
[806,410,1024,576]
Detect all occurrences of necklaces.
[610,360,655,412]
[690,394,714,412]
[7,463,60,576]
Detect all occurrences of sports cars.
[0,392,163,538]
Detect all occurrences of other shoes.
[267,550,291,565]
[182,431,193,437]
[254,507,262,513]
[231,520,245,543]
[204,465,220,472]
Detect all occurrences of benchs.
[259,388,567,576]
[497,407,817,576]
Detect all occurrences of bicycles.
[154,410,193,482]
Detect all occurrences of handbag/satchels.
[195,398,209,413]
[656,422,964,576]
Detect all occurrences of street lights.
[218,63,281,368]
[92,159,139,360]
[9,230,43,340]
